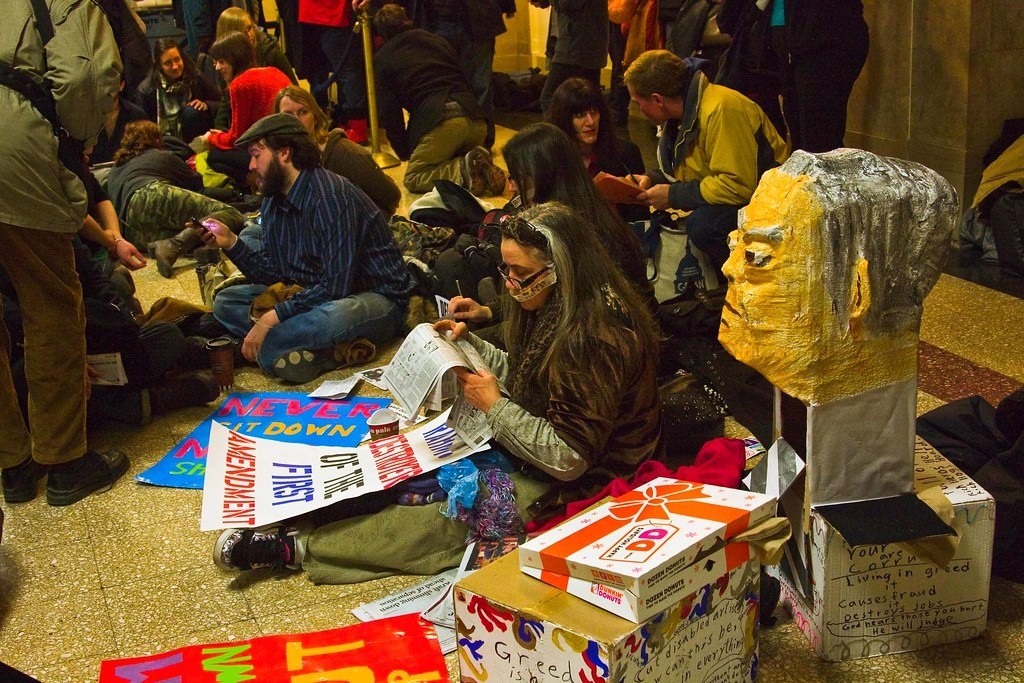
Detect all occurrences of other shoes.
[274,347,346,385]
[459,145,507,198]
[607,107,630,125]
[144,371,221,415]
[338,119,370,147]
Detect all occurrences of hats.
[233,113,310,147]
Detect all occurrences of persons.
[717,148,959,406]
[0,0,151,506]
[213,202,662,584]
[0,0,871,428]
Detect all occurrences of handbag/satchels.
[641,209,718,305]
[658,273,806,455]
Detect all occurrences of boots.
[146,225,222,278]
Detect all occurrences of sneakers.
[213,522,302,572]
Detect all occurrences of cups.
[366,408,399,441]
[205,338,235,391]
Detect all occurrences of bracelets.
[249,315,273,329]
[112,238,123,259]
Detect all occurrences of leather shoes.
[1,455,48,503]
[46,448,132,505]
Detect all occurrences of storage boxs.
[452,495,762,683]
[765,435,997,663]
[517,474,778,625]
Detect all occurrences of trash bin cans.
[140,15,187,62]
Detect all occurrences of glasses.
[497,261,550,291]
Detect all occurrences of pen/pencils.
[621,160,639,185]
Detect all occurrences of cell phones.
[191,217,216,239]
